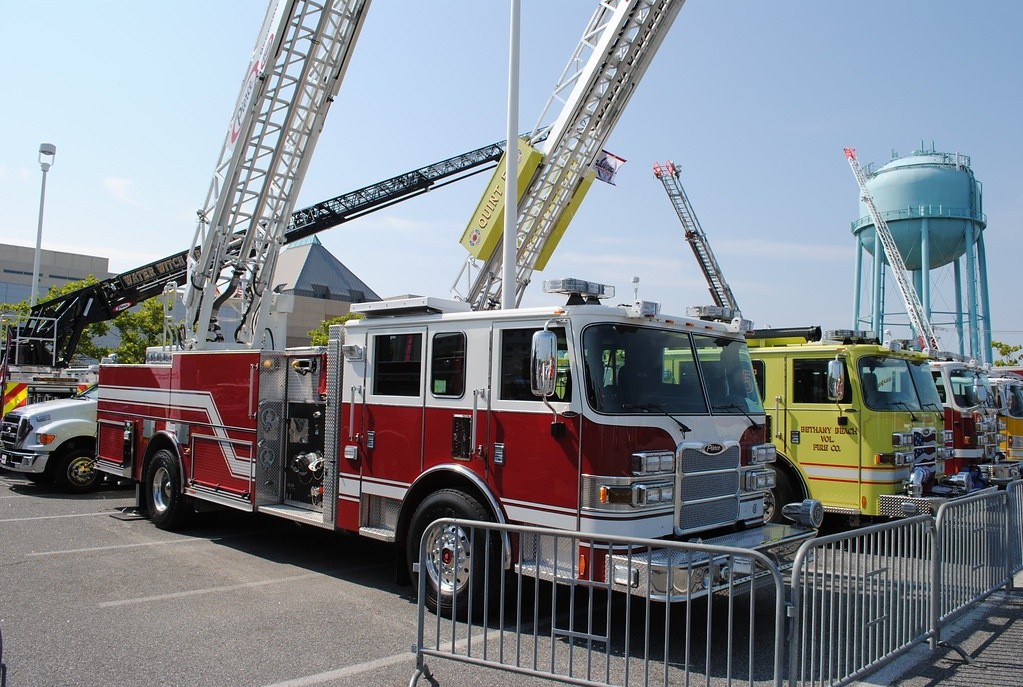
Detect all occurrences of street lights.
[632,276,640,301]
[29,143,56,311]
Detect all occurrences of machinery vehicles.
[0,0,1023,620]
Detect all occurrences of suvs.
[0,380,99,494]
